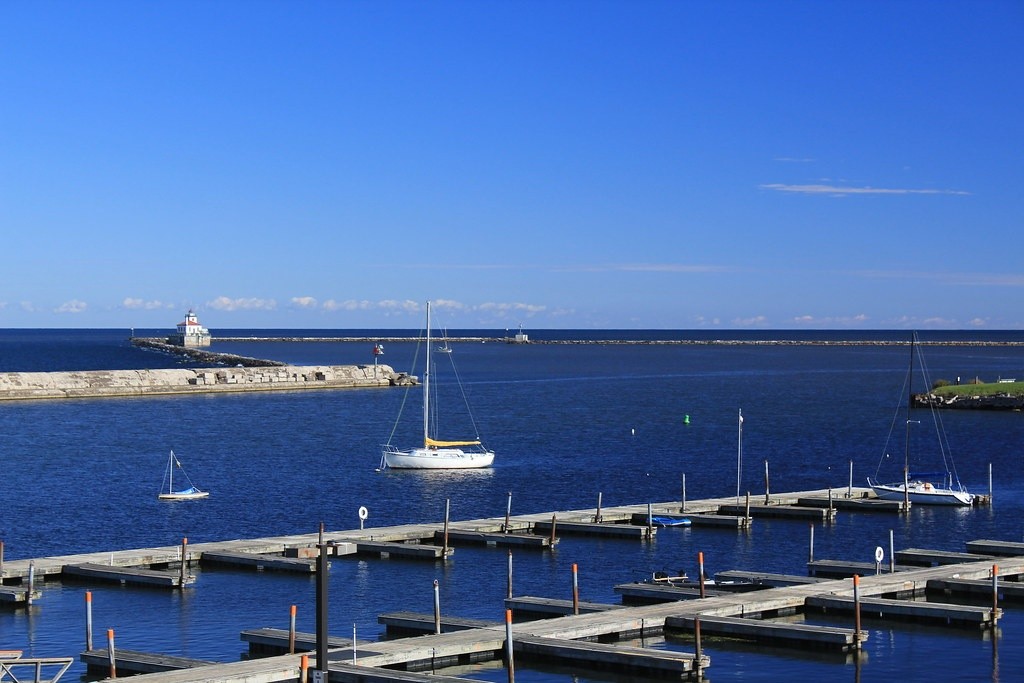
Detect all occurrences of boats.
[646,516,692,528]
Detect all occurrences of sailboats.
[377,299,496,469]
[158,449,210,502]
[866,328,974,507]
[434,326,452,354]
